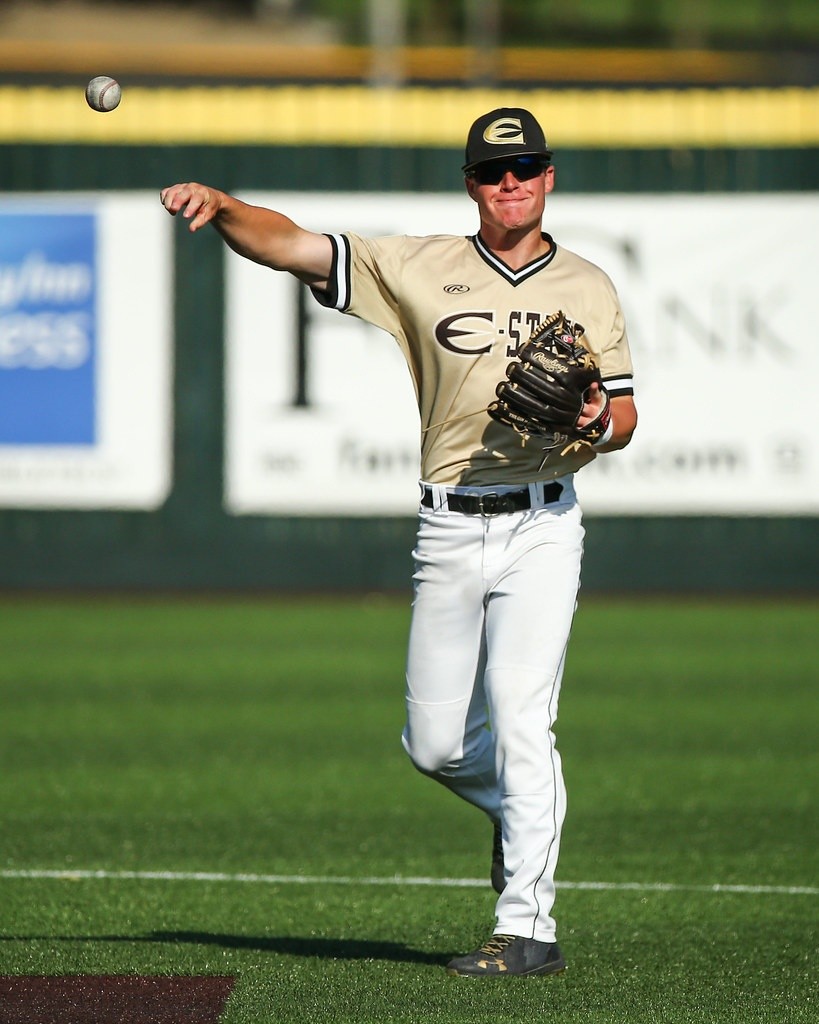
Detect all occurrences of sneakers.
[489,822,507,893]
[445,934,568,979]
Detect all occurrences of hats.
[461,108,551,176]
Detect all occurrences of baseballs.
[85,76,122,112]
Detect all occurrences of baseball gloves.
[487,311,612,447]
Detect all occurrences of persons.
[160,107,638,976]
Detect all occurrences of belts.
[421,481,563,518]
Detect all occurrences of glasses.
[470,155,551,186]
